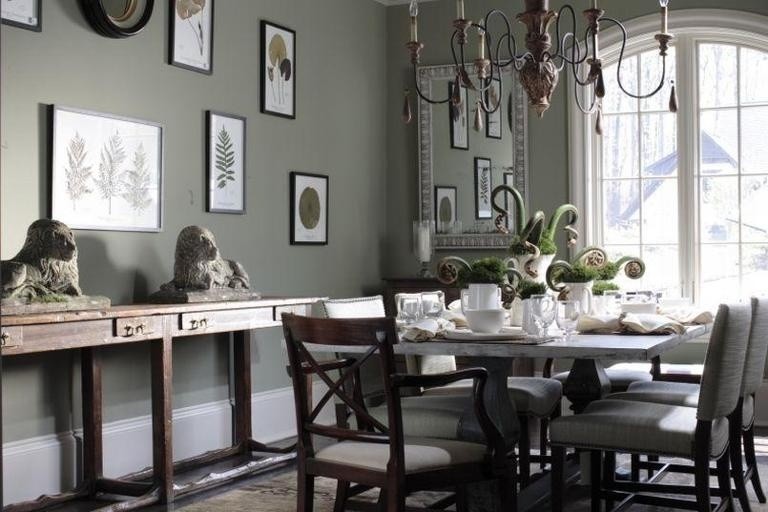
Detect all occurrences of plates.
[443,328,528,340]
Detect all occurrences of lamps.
[401,1,677,135]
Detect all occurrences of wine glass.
[529,293,556,336]
[421,291,444,317]
[555,300,580,336]
[397,295,419,318]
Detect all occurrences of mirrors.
[416,59,528,251]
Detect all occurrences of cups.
[603,290,623,315]
[522,299,541,335]
[565,280,594,319]
[461,283,498,314]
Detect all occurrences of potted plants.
[437,186,645,327]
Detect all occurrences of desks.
[381,277,536,395]
[1,298,329,512]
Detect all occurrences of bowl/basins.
[621,303,656,314]
[465,307,506,334]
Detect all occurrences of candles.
[417,226,430,262]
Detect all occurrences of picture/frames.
[205,110,246,215]
[260,19,296,119]
[434,186,457,235]
[1,0,42,33]
[475,157,493,219]
[503,173,514,232]
[46,103,165,234]
[508,92,511,132]
[289,171,329,246]
[485,77,502,139]
[168,0,214,76]
[449,81,469,150]
